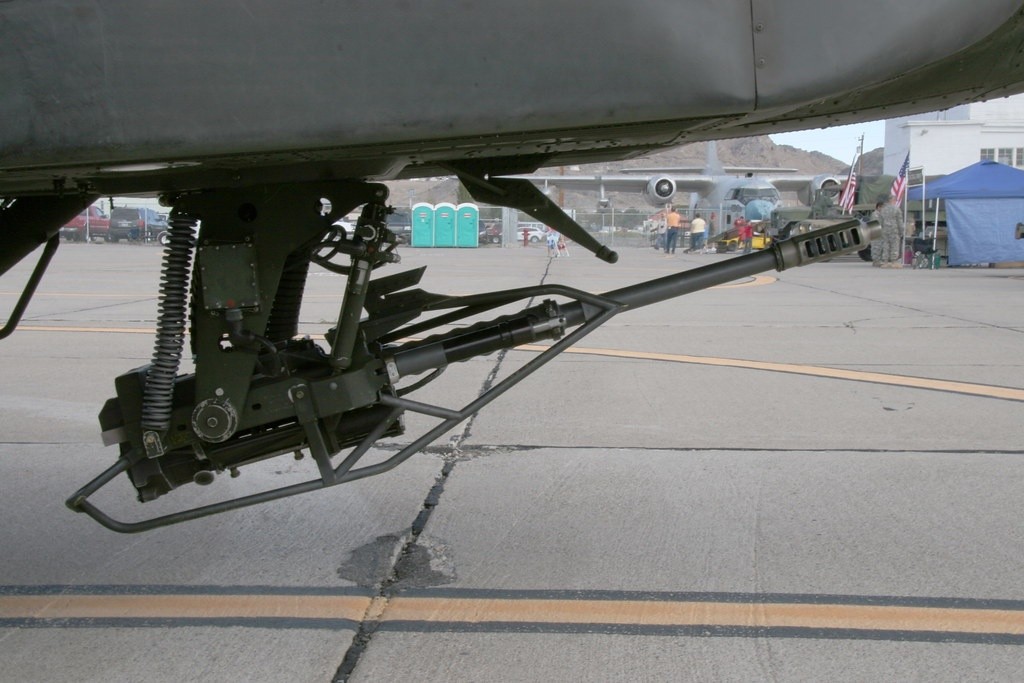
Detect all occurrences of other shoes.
[672,250,675,254]
[664,250,670,254]
[654,246,659,250]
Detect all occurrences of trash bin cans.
[410,202,479,248]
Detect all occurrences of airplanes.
[0,1,1024,537]
[490,139,851,252]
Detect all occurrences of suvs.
[58,204,111,242]
[108,206,168,243]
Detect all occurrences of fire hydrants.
[522,228,531,247]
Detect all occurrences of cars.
[479,218,548,245]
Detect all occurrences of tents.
[907,159,1024,265]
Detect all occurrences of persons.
[868,202,886,267]
[547,227,568,258]
[882,195,904,269]
[651,213,668,250]
[735,216,753,255]
[689,213,706,249]
[665,207,680,254]
[703,218,709,248]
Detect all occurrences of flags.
[838,165,857,214]
[889,153,909,208]
